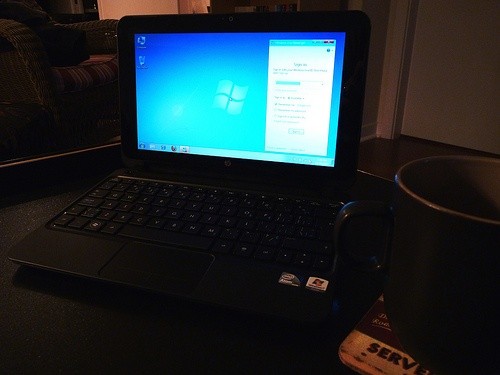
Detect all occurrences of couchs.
[0,0,119,157]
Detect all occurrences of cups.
[335,154,499,373]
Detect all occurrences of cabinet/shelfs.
[209,0,341,14]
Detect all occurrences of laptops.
[8,10,373,329]
